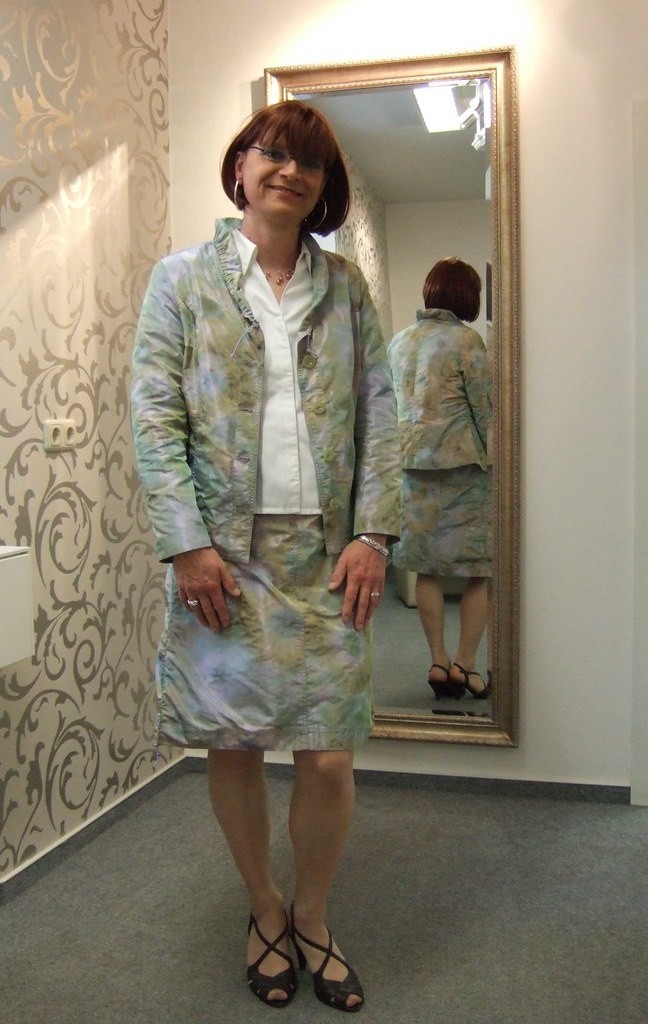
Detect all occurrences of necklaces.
[262,262,295,285]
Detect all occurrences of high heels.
[448,662,488,700]
[289,900,364,1012]
[246,908,298,1009]
[428,663,465,701]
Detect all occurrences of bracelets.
[355,534,390,557]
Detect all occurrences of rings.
[187,599,199,607]
[371,592,380,597]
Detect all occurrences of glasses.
[250,145,325,176]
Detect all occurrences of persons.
[385,256,492,702]
[131,100,404,1012]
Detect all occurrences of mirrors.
[264,45,523,746]
